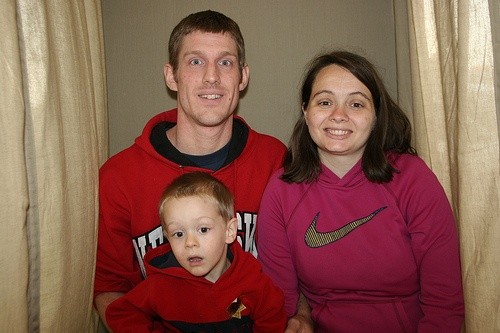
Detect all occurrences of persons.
[255,50,466,333]
[94,10,312,333]
